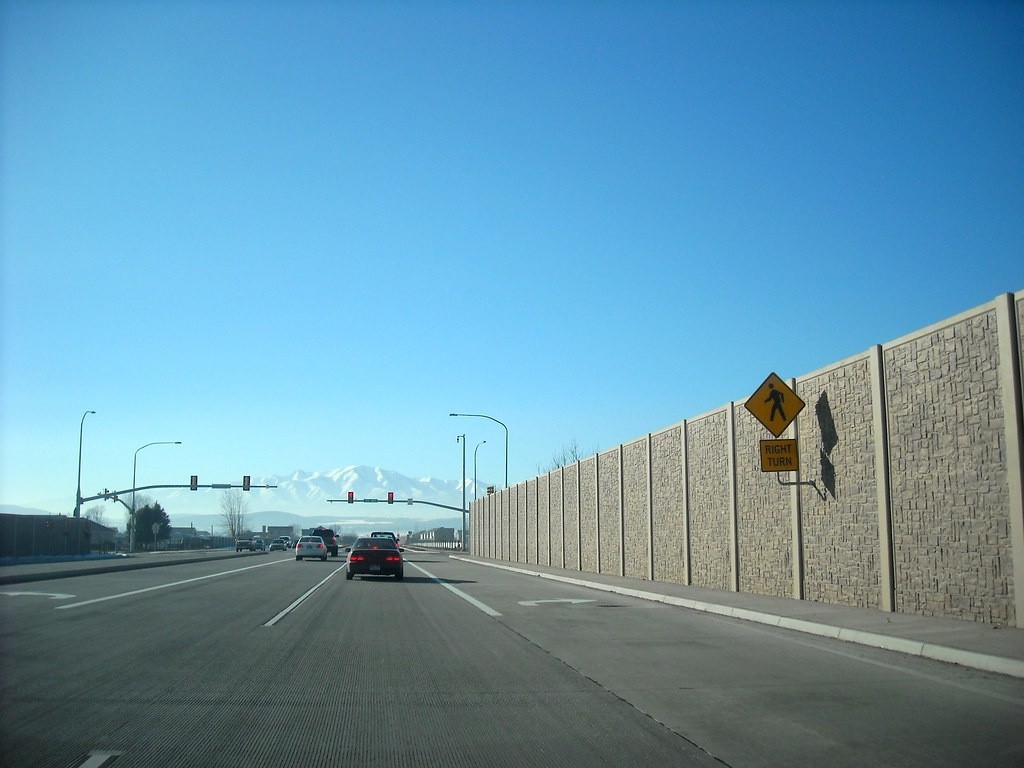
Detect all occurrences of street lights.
[475,441,486,501]
[129,441,182,553]
[76,411,96,518]
[449,413,508,491]
[457,433,466,551]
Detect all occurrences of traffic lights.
[347,491,354,504]
[388,492,393,504]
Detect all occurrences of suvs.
[309,528,340,557]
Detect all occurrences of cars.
[254,536,265,551]
[370,531,400,549]
[345,536,404,580]
[295,536,328,562]
[278,535,293,549]
[235,536,257,551]
[269,539,286,552]
